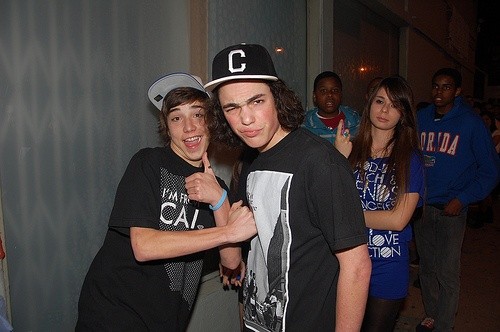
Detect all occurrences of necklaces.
[209,189,227,211]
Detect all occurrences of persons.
[203,42,371,332]
[334,75,425,332]
[364,77,385,104]
[466,95,500,224]
[303,71,362,144]
[416,67,500,332]
[76,72,258,332]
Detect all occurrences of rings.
[343,132,348,138]
[208,165,211,168]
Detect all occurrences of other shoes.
[416,317,434,332]
[409,251,419,267]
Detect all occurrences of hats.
[203,42,279,92]
[148,73,212,111]
